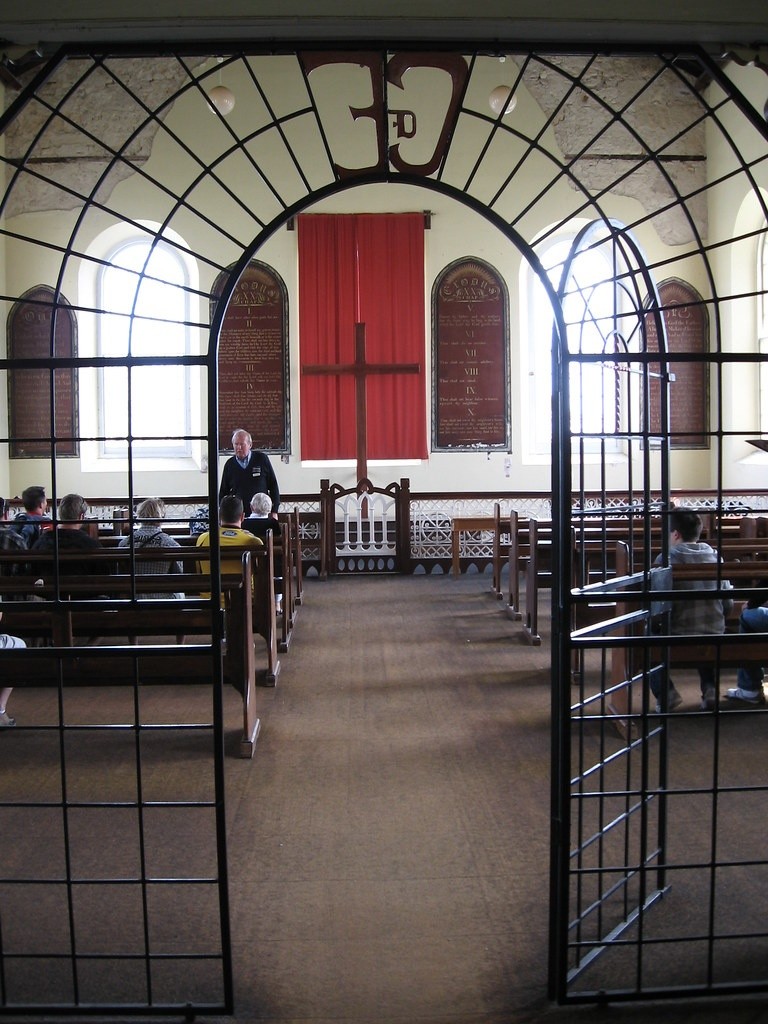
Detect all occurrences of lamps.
[209,57,235,115]
[489,53,517,115]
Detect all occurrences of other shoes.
[655,688,683,713]
[700,687,716,710]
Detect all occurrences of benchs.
[490,501,768,738]
[0,506,303,758]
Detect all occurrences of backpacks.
[8,513,52,547]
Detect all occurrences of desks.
[450,517,528,578]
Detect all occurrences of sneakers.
[727,688,764,704]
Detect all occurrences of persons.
[195,496,266,609]
[117,498,185,645]
[32,494,113,576]
[219,429,280,521]
[726,577,768,704]
[10,486,59,550]
[0,497,27,602]
[240,493,282,616]
[189,504,220,536]
[646,508,733,714]
